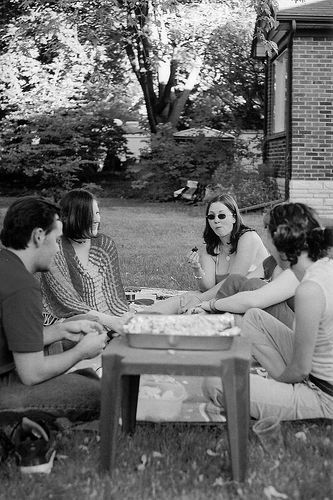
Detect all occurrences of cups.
[253,416,286,458]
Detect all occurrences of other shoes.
[9,416,56,474]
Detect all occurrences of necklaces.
[221,248,234,262]
[71,239,89,244]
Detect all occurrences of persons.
[0,195,107,461]
[177,201,333,331]
[202,203,333,421]
[38,189,134,354]
[135,193,274,314]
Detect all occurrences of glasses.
[206,214,236,219]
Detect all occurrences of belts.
[308,373,333,397]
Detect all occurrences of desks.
[99,334,252,483]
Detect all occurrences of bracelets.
[193,271,206,280]
[208,298,220,312]
